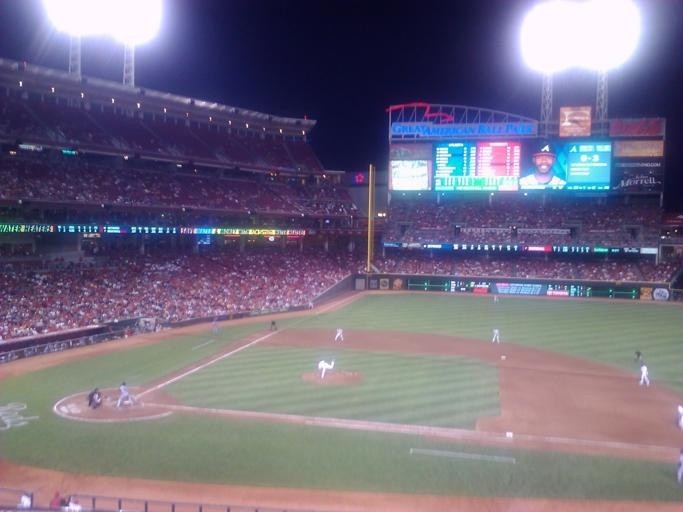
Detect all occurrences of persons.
[0,238,366,342]
[675,448,683,486]
[90,391,104,408]
[0,98,167,152]
[676,403,682,429]
[60,496,68,511]
[18,490,31,508]
[87,386,98,407]
[0,148,361,216]
[49,490,62,508]
[639,363,649,385]
[516,138,567,193]
[67,494,81,512]
[492,328,500,343]
[369,198,682,287]
[317,359,337,378]
[634,350,642,361]
[115,381,137,410]
